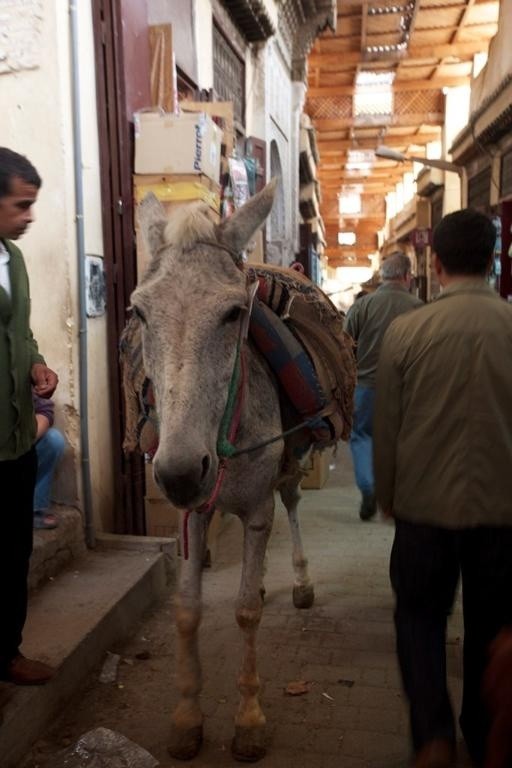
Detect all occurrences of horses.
[126,177,362,763]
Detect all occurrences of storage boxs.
[131,107,225,238]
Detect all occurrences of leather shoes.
[410,735,456,768]
[2,655,56,685]
[360,497,378,520]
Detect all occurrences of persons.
[0,143,60,684]
[342,248,423,520]
[368,205,511,767]
[27,385,64,528]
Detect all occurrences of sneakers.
[33,509,59,528]
